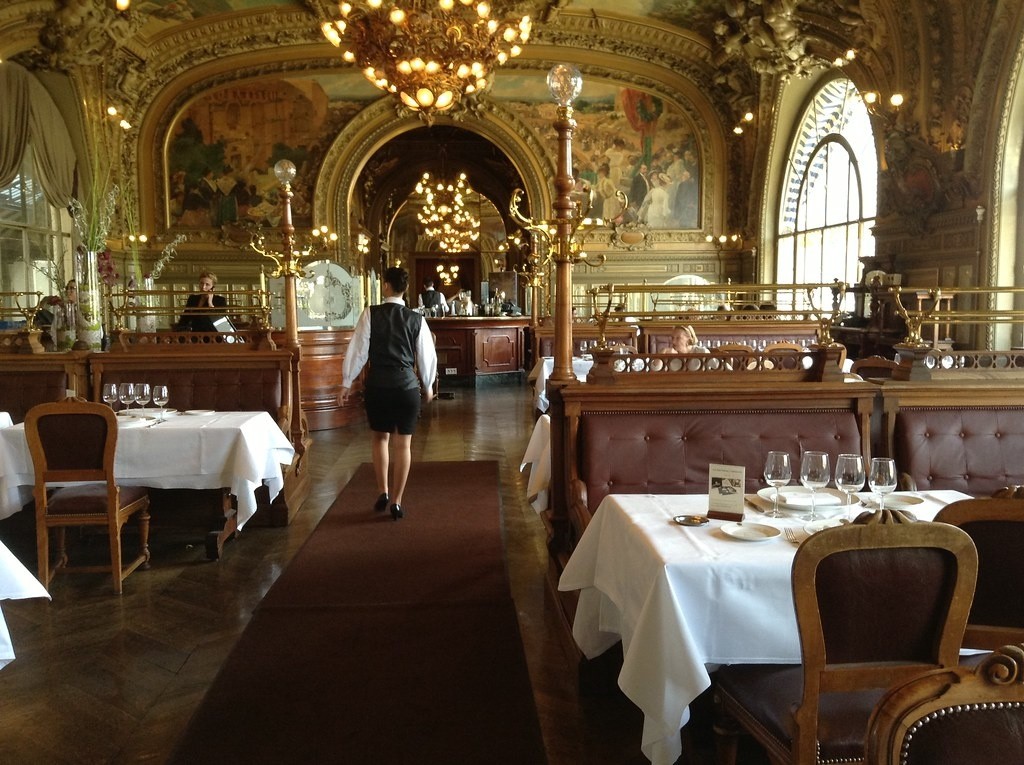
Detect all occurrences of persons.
[179,270,227,343]
[65,279,76,304]
[336,267,437,521]
[418,278,466,317]
[572,303,776,323]
[661,324,719,371]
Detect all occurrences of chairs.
[706,476,1024,765]
[24,396,152,596]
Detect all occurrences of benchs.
[0,341,313,528]
[536,320,1024,669]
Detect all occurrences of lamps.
[415,142,481,287]
[320,0,534,114]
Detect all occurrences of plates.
[119,408,177,414]
[721,522,782,541]
[185,410,215,415]
[117,416,157,426]
[673,516,710,526]
[869,494,923,508]
[757,486,861,512]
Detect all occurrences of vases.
[58,300,76,351]
[75,249,102,353]
[133,278,157,344]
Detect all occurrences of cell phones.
[211,286,214,290]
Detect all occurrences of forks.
[784,527,803,544]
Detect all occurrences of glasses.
[65,286,77,290]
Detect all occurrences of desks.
[0,410,296,563]
[830,326,908,359]
[558,490,976,765]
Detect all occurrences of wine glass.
[103,383,118,408]
[134,383,151,408]
[834,454,865,513]
[800,450,831,521]
[868,457,898,509]
[580,340,587,361]
[152,386,168,408]
[764,450,792,518]
[118,383,134,408]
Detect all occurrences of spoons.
[803,526,818,535]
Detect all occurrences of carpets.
[166,459,548,765]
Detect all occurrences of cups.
[620,347,629,354]
[590,340,616,349]
[697,340,814,349]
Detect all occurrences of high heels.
[390,504,404,520]
[374,493,390,512]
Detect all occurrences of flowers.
[66,137,118,288]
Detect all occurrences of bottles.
[413,288,502,317]
